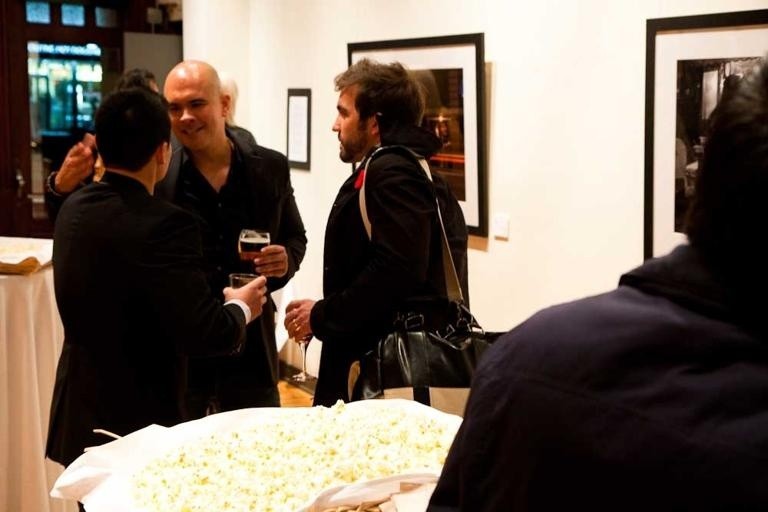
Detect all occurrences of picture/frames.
[287,88,311,170]
[346,33,488,239]
[644,7,768,263]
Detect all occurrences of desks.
[0,268,78,512]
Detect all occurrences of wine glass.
[292,337,314,385]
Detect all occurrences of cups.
[238,228,270,264]
[230,274,259,290]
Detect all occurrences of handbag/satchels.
[346,309,492,419]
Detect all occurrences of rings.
[293,319,297,327]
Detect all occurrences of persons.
[44,60,308,429]
[43,89,267,512]
[285,58,470,407]
[425,53,767,510]
[115,68,160,94]
